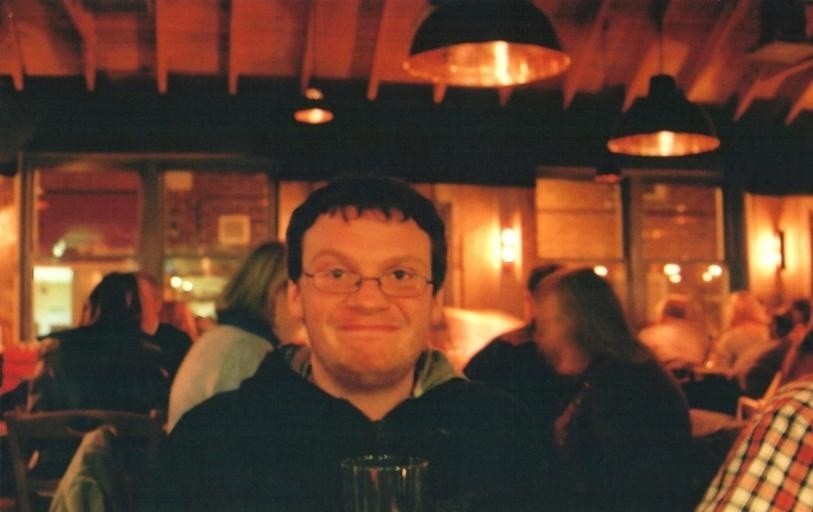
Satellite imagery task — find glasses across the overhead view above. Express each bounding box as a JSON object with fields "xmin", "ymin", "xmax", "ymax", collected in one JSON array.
[{"xmin": 300, "ymin": 266, "xmax": 432, "ymax": 299}]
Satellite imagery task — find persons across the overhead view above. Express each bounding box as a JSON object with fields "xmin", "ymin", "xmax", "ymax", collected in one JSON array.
[
  {"xmin": 145, "ymin": 177, "xmax": 570, "ymax": 509},
  {"xmin": 24, "ymin": 237, "xmax": 305, "ymax": 479},
  {"xmin": 463, "ymin": 260, "xmax": 813, "ymax": 510}
]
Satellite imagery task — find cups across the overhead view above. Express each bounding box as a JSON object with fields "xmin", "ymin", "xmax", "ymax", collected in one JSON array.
[{"xmin": 339, "ymin": 453, "xmax": 428, "ymax": 512}]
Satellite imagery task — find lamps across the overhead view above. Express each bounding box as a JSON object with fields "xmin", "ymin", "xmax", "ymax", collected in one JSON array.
[
  {"xmin": 291, "ymin": 1, "xmax": 336, "ymax": 126},
  {"xmin": 605, "ymin": 1, "xmax": 723, "ymax": 160},
  {"xmin": 404, "ymin": 0, "xmax": 570, "ymax": 88}
]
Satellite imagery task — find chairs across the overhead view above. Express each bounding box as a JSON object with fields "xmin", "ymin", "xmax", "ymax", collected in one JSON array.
[{"xmin": 3, "ymin": 399, "xmax": 169, "ymax": 512}]
